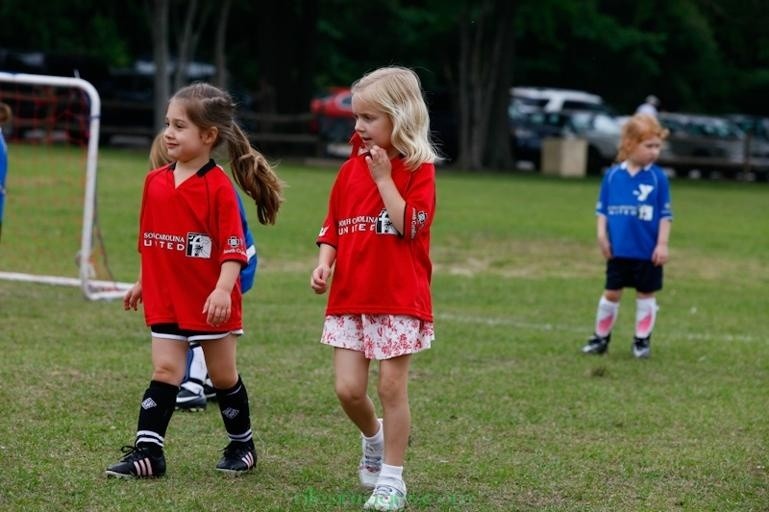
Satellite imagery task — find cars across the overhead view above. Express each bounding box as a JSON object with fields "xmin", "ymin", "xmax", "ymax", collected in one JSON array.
[
  {"xmin": 508, "ymin": 84, "xmax": 769, "ymax": 183},
  {"xmin": 309, "ymin": 85, "xmax": 358, "ymax": 136}
]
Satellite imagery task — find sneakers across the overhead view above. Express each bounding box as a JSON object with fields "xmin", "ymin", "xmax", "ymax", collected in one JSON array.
[
  {"xmin": 632, "ymin": 333, "xmax": 652, "ymax": 357},
  {"xmin": 214, "ymin": 437, "xmax": 258, "ymax": 473},
  {"xmin": 104, "ymin": 444, "xmax": 168, "ymax": 482},
  {"xmin": 580, "ymin": 331, "xmax": 611, "ymax": 354},
  {"xmin": 202, "ymin": 383, "xmax": 216, "ymax": 399},
  {"xmin": 357, "ymin": 416, "xmax": 385, "ymax": 489},
  {"xmin": 172, "ymin": 386, "xmax": 207, "ymax": 412},
  {"xmin": 363, "ymin": 474, "xmax": 406, "ymax": 512}
]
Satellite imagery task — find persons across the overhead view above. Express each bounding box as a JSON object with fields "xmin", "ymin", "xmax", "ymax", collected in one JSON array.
[
  {"xmin": 308, "ymin": 67, "xmax": 446, "ymax": 512},
  {"xmin": 578, "ymin": 118, "xmax": 674, "ymax": 358},
  {"xmin": 146, "ymin": 127, "xmax": 256, "ymax": 408},
  {"xmin": 104, "ymin": 82, "xmax": 283, "ymax": 478},
  {"xmin": 0, "ymin": 102, "xmax": 12, "ymax": 238},
  {"xmin": 636, "ymin": 96, "xmax": 658, "ymax": 117}
]
